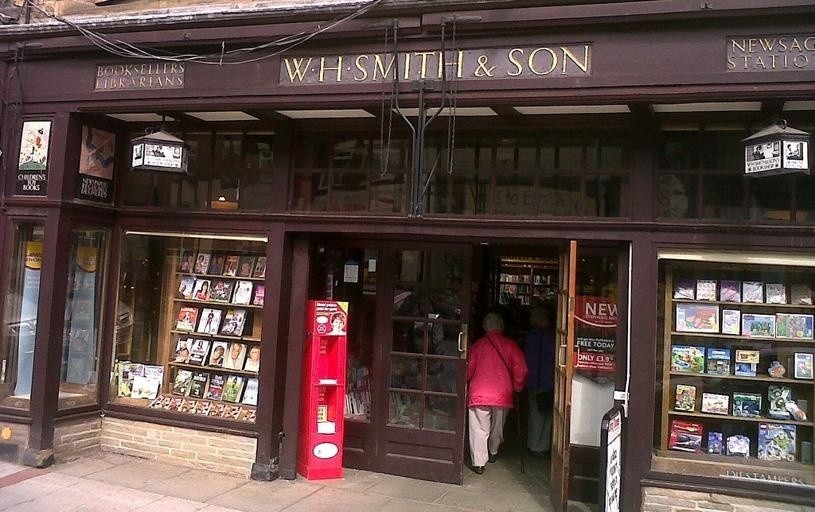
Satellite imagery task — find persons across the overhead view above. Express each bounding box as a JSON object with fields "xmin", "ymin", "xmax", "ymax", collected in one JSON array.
[
  {"xmin": 524, "ymin": 316, "xmax": 555, "ymax": 463},
  {"xmin": 327, "ymin": 312, "xmax": 346, "ymax": 334},
  {"xmin": 467, "ymin": 314, "xmax": 529, "ymax": 474}
]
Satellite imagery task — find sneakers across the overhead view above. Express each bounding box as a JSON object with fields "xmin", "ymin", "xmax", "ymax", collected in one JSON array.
[{"xmin": 531, "ymin": 450, "xmax": 549, "ymax": 458}]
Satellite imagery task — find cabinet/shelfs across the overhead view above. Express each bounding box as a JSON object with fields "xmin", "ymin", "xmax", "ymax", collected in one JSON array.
[
  {"xmin": 657, "ymin": 265, "xmax": 815, "ymax": 472},
  {"xmin": 489, "ymin": 257, "xmax": 559, "ymax": 330},
  {"xmin": 161, "ymin": 242, "xmax": 267, "ymax": 408}
]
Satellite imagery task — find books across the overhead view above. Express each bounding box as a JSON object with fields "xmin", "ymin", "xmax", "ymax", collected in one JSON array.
[
  {"xmin": 666, "ymin": 277, "xmax": 815, "ymax": 462},
  {"xmin": 112, "ymin": 238, "xmax": 268, "ymax": 423},
  {"xmin": 345, "ymin": 365, "xmax": 373, "ymax": 418}
]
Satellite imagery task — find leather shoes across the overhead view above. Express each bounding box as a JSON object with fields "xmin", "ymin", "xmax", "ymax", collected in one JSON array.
[
  {"xmin": 471, "ymin": 466, "xmax": 484, "ymax": 474},
  {"xmin": 489, "ymin": 449, "xmax": 501, "ymax": 463}
]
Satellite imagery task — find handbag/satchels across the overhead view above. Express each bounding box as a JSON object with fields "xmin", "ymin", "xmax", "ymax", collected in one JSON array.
[{"xmin": 536, "ymin": 391, "xmax": 554, "ymax": 412}]
